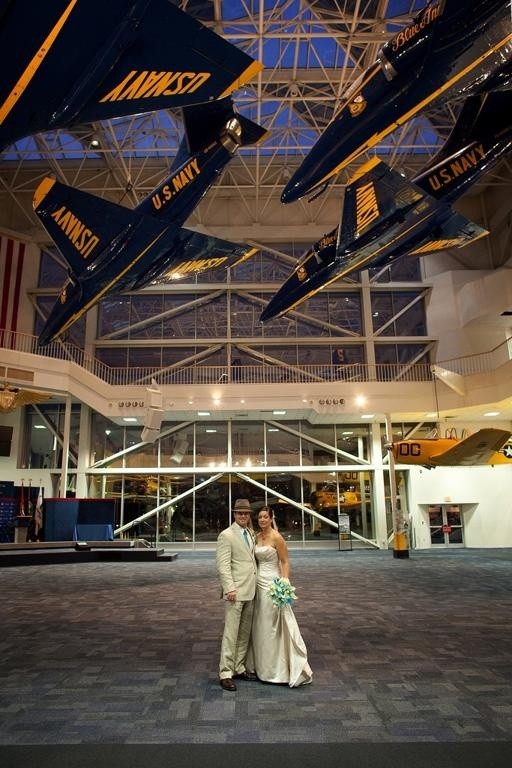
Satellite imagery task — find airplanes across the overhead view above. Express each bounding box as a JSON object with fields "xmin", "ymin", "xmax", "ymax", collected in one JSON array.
[
  {"xmin": 257, "ymin": 58, "xmax": 512, "ymax": 324},
  {"xmin": 306, "ymin": 489, "xmax": 400, "ymax": 512},
  {"xmin": 102, "ymin": 480, "xmax": 220, "ymax": 503},
  {"xmin": 0, "ymin": 1, "xmax": 266, "ymax": 149},
  {"xmin": 279, "ymin": 0, "xmax": 512, "ymax": 218},
  {"xmin": 383, "ymin": 427, "xmax": 512, "ymax": 467},
  {"xmin": 30, "ymin": 101, "xmax": 267, "ymax": 348}
]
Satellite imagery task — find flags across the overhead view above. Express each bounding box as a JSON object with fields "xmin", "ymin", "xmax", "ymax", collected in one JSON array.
[
  {"xmin": 34, "ymin": 486, "xmax": 44, "ymax": 529},
  {"xmin": 20, "ymin": 489, "xmax": 25, "ymax": 517},
  {"xmin": 28, "ymin": 498, "xmax": 35, "ymax": 522}
]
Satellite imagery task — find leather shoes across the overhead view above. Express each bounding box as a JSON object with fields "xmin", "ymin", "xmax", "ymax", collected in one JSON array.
[
  {"xmin": 232, "ymin": 671, "xmax": 257, "ymax": 681},
  {"xmin": 220, "ymin": 679, "xmax": 237, "ymax": 691}
]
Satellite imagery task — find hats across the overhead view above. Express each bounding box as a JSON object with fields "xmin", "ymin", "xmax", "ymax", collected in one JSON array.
[{"xmin": 231, "ymin": 499, "xmax": 253, "ymax": 513}]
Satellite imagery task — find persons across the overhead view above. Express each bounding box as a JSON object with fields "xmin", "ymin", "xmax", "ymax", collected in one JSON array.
[
  {"xmin": 253, "ymin": 507, "xmax": 313, "ymax": 687},
  {"xmin": 214, "ymin": 498, "xmax": 258, "ymax": 692}
]
